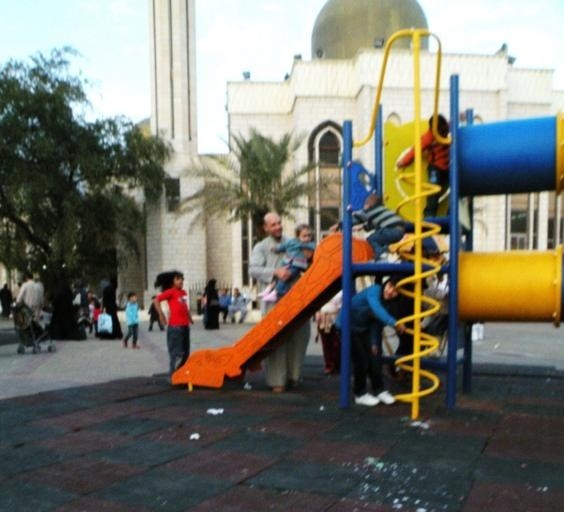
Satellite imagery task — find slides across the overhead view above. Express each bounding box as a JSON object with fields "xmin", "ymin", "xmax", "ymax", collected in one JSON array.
[{"xmin": 171, "ymin": 233, "xmax": 372, "ymax": 388}]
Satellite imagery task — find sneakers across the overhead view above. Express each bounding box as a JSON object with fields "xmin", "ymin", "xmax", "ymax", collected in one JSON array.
[
  {"xmin": 377, "ymin": 390, "xmax": 395, "ymax": 405},
  {"xmin": 354, "ymin": 394, "xmax": 379, "ymax": 406},
  {"xmin": 378, "ymin": 251, "xmax": 403, "ymax": 265}
]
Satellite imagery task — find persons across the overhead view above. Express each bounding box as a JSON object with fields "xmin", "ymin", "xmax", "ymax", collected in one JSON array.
[
  {"xmin": 0, "ymin": 271, "xmax": 247, "ymax": 377},
  {"xmin": 249, "ymin": 212, "xmax": 311, "ymax": 393},
  {"xmin": 331, "ymin": 195, "xmax": 405, "ymax": 264},
  {"xmin": 314, "ymin": 289, "xmax": 343, "ymax": 375},
  {"xmin": 352, "ymin": 277, "xmax": 406, "ymax": 407},
  {"xmin": 395, "ymin": 116, "xmax": 450, "ymax": 219},
  {"xmin": 256, "ymin": 225, "xmax": 316, "ymax": 302}
]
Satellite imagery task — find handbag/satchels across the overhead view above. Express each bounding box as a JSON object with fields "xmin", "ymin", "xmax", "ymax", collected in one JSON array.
[{"xmin": 96, "ymin": 313, "xmax": 114, "ymax": 336}]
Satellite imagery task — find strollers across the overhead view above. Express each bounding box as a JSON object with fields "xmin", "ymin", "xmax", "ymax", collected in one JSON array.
[{"xmin": 9, "ymin": 300, "xmax": 58, "ymax": 354}]
